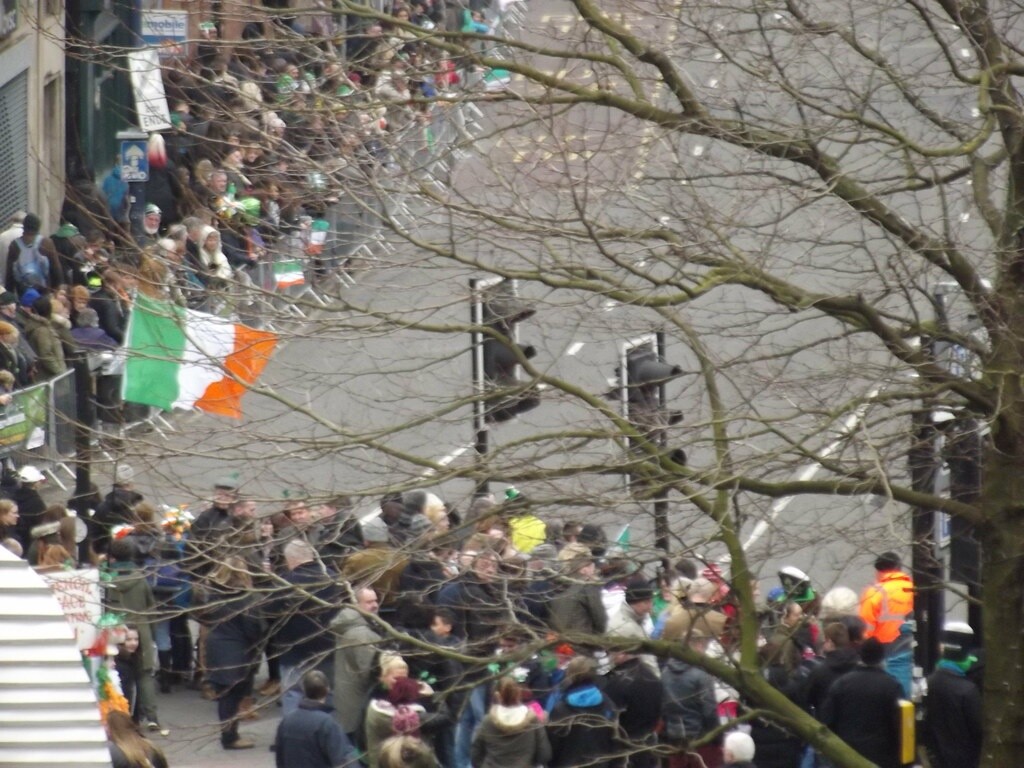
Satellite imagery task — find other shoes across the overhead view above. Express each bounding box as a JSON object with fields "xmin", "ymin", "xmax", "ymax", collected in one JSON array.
[
  {"xmin": 222, "ymin": 734, "xmax": 254, "ymax": 749},
  {"xmin": 148, "ymin": 719, "xmax": 161, "ymax": 730},
  {"xmin": 200, "ymin": 682, "xmax": 216, "ymax": 699},
  {"xmin": 261, "ymin": 675, "xmax": 281, "ymax": 695}
]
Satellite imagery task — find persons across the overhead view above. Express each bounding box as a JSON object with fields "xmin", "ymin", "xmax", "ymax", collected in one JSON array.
[
  {"xmin": 107, "ymin": 710, "xmax": 170, "ymax": 768},
  {"xmin": 0, "ymin": 131, "xmax": 227, "ymax": 403},
  {"xmin": 0, "ymin": 464, "xmax": 985, "ymax": 768},
  {"xmin": 115, "ymin": 7, "xmax": 490, "ymax": 276}
]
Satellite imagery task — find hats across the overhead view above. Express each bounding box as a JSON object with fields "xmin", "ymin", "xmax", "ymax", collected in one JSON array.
[
  {"xmin": 388, "ymin": 676, "xmax": 417, "ymax": 703},
  {"xmin": 19, "ymin": 465, "xmax": 46, "ymax": 484},
  {"xmin": 818, "ymin": 587, "xmax": 859, "ymax": 619},
  {"xmin": 32, "ymin": 298, "xmax": 51, "ymax": 313},
  {"xmin": 212, "ymin": 476, "xmax": 238, "ymax": 492},
  {"xmin": 625, "ymin": 577, "xmax": 652, "ymax": 603},
  {"xmin": 69, "ymin": 285, "xmax": 90, "ymax": 300},
  {"xmin": 687, "ymin": 577, "xmax": 713, "ymax": 604},
  {"xmin": 113, "ymin": 464, "xmax": 133, "ymax": 485},
  {"xmin": 391, "ymin": 705, "xmax": 420, "ymax": 737},
  {"xmin": 21, "ymin": 288, "xmax": 41, "ymax": 307},
  {"xmin": 0, "ymin": 292, "xmax": 20, "ymax": 305}
]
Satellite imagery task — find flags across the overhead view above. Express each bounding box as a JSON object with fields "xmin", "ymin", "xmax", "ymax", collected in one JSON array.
[
  {"xmin": 303, "ymin": 219, "xmax": 330, "ymax": 256},
  {"xmin": 270, "ymin": 261, "xmax": 306, "ymax": 288},
  {"xmin": 118, "ymin": 290, "xmax": 279, "ymax": 420}
]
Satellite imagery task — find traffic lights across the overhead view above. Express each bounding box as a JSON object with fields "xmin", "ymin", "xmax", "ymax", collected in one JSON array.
[
  {"xmin": 623, "ymin": 334, "xmax": 688, "ymax": 501},
  {"xmin": 471, "ymin": 276, "xmax": 542, "ymax": 429}
]
